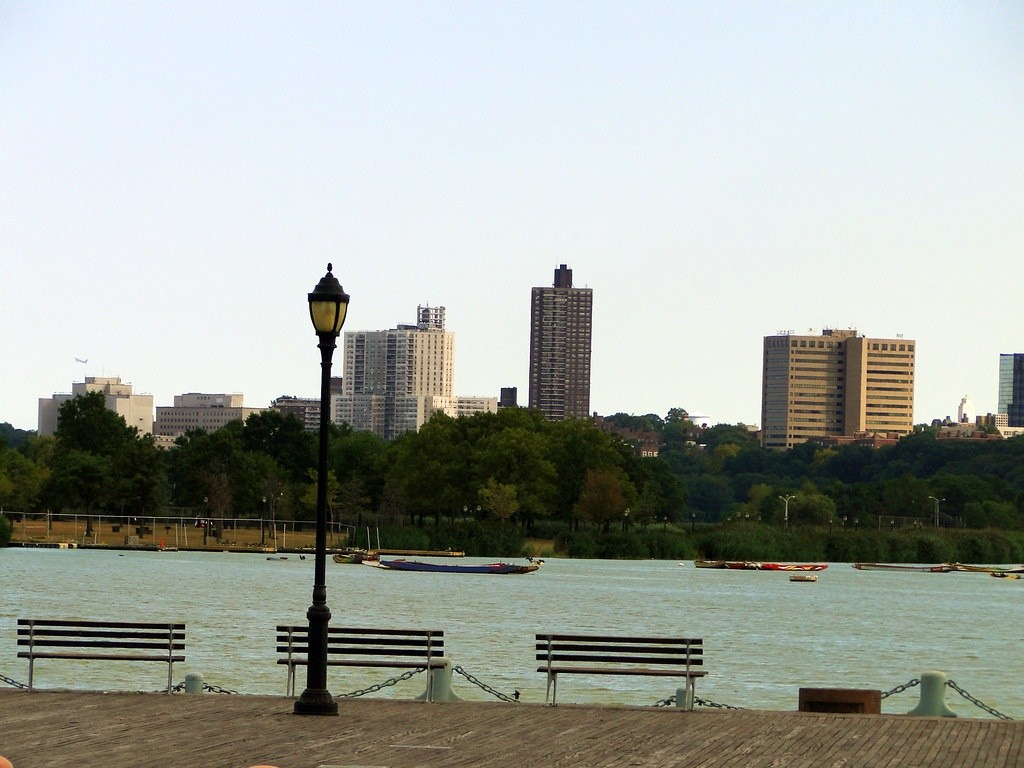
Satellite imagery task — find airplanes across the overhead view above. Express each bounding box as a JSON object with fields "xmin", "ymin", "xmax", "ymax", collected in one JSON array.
[{"xmin": 75, "ymin": 358, "xmax": 88, "ymax": 363}]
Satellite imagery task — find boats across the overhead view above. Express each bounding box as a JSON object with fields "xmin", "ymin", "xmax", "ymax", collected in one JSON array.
[
  {"xmin": 990, "ymin": 572, "xmax": 1021, "ymax": 579},
  {"xmin": 852, "ymin": 564, "xmax": 954, "ymax": 573},
  {"xmin": 950, "ymin": 564, "xmax": 1023, "ymax": 573},
  {"xmin": 694, "ymin": 560, "xmax": 828, "ymax": 570},
  {"xmin": 378, "ymin": 559, "xmax": 539, "ymax": 574},
  {"xmin": 353, "ymin": 551, "xmax": 378, "ymax": 560},
  {"xmin": 789, "ymin": 576, "xmax": 818, "ymax": 582},
  {"xmin": 333, "ymin": 555, "xmax": 363, "ymax": 564}
]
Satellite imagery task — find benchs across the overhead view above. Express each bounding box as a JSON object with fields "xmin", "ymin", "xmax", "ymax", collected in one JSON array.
[
  {"xmin": 14, "ymin": 619, "xmax": 186, "ymax": 694},
  {"xmin": 534, "ymin": 631, "xmax": 707, "ymax": 711},
  {"xmin": 274, "ymin": 624, "xmax": 445, "ymax": 702}
]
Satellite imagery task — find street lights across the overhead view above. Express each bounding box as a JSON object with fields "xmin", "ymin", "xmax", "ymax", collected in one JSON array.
[
  {"xmin": 262, "ymin": 496, "xmax": 267, "ymax": 544},
  {"xmin": 919, "ymin": 521, "xmax": 922, "ymax": 531},
  {"xmin": 855, "ymin": 519, "xmax": 858, "ymax": 531},
  {"xmin": 779, "ymin": 496, "xmax": 795, "ymax": 528},
  {"xmin": 663, "ymin": 515, "xmax": 667, "ymax": 527},
  {"xmin": 758, "ymin": 514, "xmax": 761, "ymax": 523},
  {"xmin": 745, "ymin": 512, "xmax": 749, "ymax": 521},
  {"xmin": 692, "ymin": 513, "xmax": 695, "ymax": 527},
  {"xmin": 463, "ymin": 505, "xmax": 467, "ymax": 522},
  {"xmin": 844, "ymin": 516, "xmax": 847, "ymax": 532},
  {"xmin": 204, "ymin": 496, "xmax": 208, "ymax": 545},
  {"xmin": 292, "ymin": 263, "xmax": 350, "ymax": 715},
  {"xmin": 727, "ymin": 516, "xmax": 730, "ymax": 523},
  {"xmin": 737, "ymin": 511, "xmax": 740, "ymax": 521},
  {"xmin": 913, "ymin": 520, "xmax": 917, "ymax": 528},
  {"xmin": 891, "ymin": 520, "xmax": 894, "ymax": 530},
  {"xmin": 624, "ymin": 510, "xmax": 628, "ymax": 530},
  {"xmin": 477, "ymin": 505, "xmax": 481, "ymax": 520},
  {"xmin": 929, "ymin": 496, "xmax": 945, "ymax": 528},
  {"xmin": 829, "ymin": 519, "xmax": 832, "ymax": 533}
]
